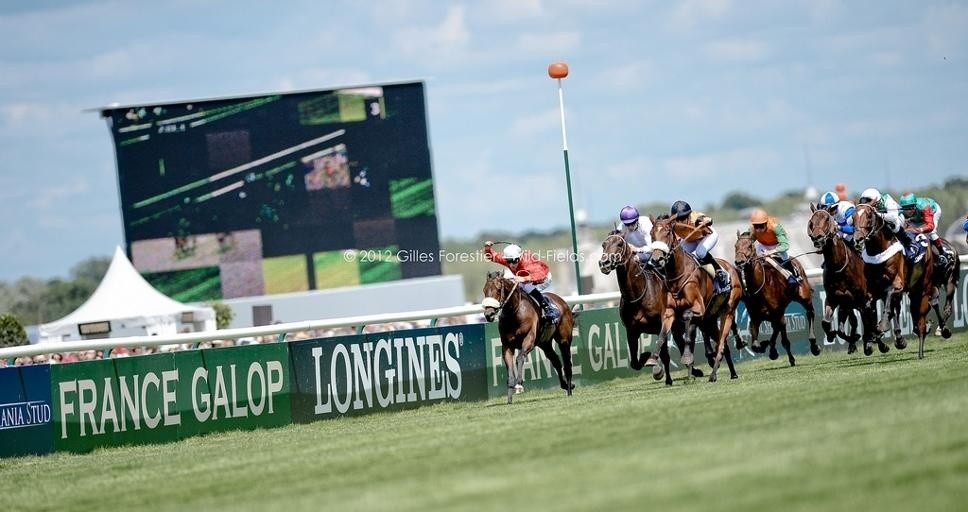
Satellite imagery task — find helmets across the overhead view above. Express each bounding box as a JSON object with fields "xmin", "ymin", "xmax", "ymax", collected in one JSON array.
[
  {"xmin": 820, "ymin": 192, "xmax": 840, "ymax": 206},
  {"xmin": 900, "ymin": 192, "xmax": 917, "ymax": 207},
  {"xmin": 862, "ymin": 189, "xmax": 881, "ymax": 205},
  {"xmin": 502, "ymin": 244, "xmax": 522, "ymax": 258},
  {"xmin": 671, "ymin": 201, "xmax": 692, "ymax": 217},
  {"xmin": 751, "ymin": 210, "xmax": 767, "ymax": 224},
  {"xmin": 620, "ymin": 206, "xmax": 639, "ymax": 224}
]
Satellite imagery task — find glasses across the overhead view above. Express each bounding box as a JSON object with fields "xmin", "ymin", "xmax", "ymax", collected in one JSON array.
[
  {"xmin": 506, "ymin": 258, "xmax": 519, "ymax": 264},
  {"xmin": 753, "ymin": 224, "xmax": 764, "ymax": 228},
  {"xmin": 859, "ymin": 198, "xmax": 871, "ymax": 202},
  {"xmin": 624, "ymin": 221, "xmax": 636, "ymax": 227}
]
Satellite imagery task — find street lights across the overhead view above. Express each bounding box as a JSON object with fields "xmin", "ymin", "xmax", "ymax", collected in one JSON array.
[{"xmin": 545, "ymin": 58, "xmax": 587, "ymax": 310}]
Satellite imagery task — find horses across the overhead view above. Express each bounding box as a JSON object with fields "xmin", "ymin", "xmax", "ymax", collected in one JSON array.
[
  {"xmin": 735, "ymin": 225, "xmax": 822, "ymax": 366},
  {"xmin": 483, "ymin": 269, "xmax": 583, "ymax": 405},
  {"xmin": 596, "ymin": 226, "xmax": 747, "ymax": 384},
  {"xmin": 806, "ymin": 202, "xmax": 961, "ymax": 358}
]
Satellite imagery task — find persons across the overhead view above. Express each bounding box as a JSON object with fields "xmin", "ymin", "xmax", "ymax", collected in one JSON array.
[
  {"xmin": 670, "ymin": 201, "xmax": 731, "ymax": 290},
  {"xmin": 899, "ymin": 191, "xmax": 949, "ymax": 266},
  {"xmin": 484, "ymin": 241, "xmax": 561, "ymax": 322},
  {"xmin": 858, "ymin": 188, "xmax": 920, "ymax": 259},
  {"xmin": 816, "ymin": 192, "xmax": 856, "ymax": 246},
  {"xmin": 748, "ymin": 208, "xmax": 803, "ymax": 283},
  {"xmin": 0, "ymin": 312, "xmax": 490, "ymax": 370},
  {"xmin": 608, "ymin": 206, "xmax": 654, "ymax": 263}
]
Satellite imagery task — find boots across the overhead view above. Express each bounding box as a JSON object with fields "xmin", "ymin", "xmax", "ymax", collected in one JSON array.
[
  {"xmin": 932, "ymin": 238, "xmax": 948, "ymax": 267},
  {"xmin": 702, "ymin": 252, "xmax": 727, "ymax": 289},
  {"xmin": 529, "ymin": 288, "xmax": 553, "ymax": 321},
  {"xmin": 784, "ymin": 259, "xmax": 803, "ymax": 284}
]
